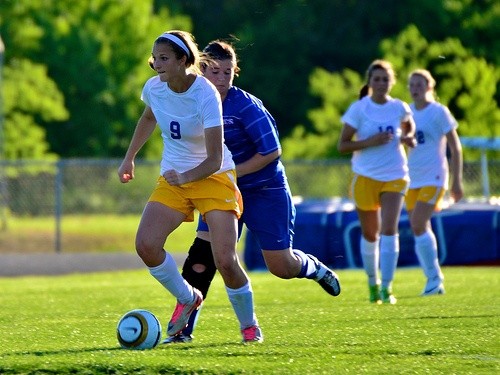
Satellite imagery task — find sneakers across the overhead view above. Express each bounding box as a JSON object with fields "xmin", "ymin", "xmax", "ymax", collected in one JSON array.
[
  {"xmin": 241, "ymin": 326, "xmax": 263, "ymax": 342},
  {"xmin": 166, "ymin": 287, "xmax": 203, "ymax": 335},
  {"xmin": 307, "ymin": 253, "xmax": 340, "ymax": 296},
  {"xmin": 164, "ymin": 334, "xmax": 192, "ymax": 343}
]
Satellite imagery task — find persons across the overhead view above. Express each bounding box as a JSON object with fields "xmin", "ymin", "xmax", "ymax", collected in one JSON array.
[
  {"xmin": 118, "ymin": 30, "xmax": 265, "ymax": 343},
  {"xmin": 405, "ymin": 70, "xmax": 464, "ymax": 295},
  {"xmin": 338, "ymin": 60, "xmax": 417, "ymax": 304},
  {"xmin": 163, "ymin": 41, "xmax": 341, "ymax": 342}
]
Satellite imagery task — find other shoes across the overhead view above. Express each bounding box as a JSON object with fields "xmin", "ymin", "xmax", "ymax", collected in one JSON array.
[
  {"xmin": 423, "ymin": 283, "xmax": 444, "ymax": 295},
  {"xmin": 370, "ymin": 282, "xmax": 383, "ymax": 304},
  {"xmin": 381, "ymin": 283, "xmax": 396, "ymax": 304}
]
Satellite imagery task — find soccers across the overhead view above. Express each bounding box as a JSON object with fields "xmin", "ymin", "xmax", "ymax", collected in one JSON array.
[{"xmin": 116, "ymin": 309, "xmax": 162, "ymax": 350}]
